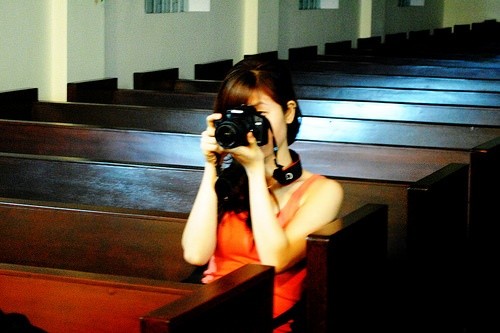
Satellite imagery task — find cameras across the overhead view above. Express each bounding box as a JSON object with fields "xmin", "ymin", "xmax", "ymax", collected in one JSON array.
[{"xmin": 213, "ymin": 104, "xmax": 270, "ymax": 150}]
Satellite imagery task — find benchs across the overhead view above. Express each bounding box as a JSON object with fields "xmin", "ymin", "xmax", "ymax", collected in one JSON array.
[{"xmin": 1, "ymin": 24, "xmax": 500, "ymax": 333}]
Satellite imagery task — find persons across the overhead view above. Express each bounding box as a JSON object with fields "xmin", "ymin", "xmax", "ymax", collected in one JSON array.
[{"xmin": 180, "ymin": 59, "xmax": 345, "ymax": 333}]
